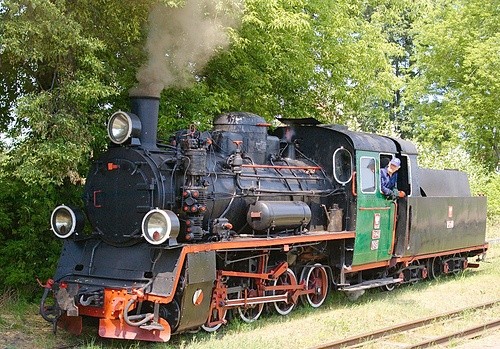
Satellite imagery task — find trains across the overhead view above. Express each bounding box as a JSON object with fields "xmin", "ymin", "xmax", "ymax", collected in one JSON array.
[{"xmin": 38, "ymin": 94, "xmax": 489, "ymax": 344}]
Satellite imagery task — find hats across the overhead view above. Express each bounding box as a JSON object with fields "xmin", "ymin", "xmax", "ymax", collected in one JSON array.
[{"xmin": 388, "ymin": 157, "xmax": 401, "ymax": 167}]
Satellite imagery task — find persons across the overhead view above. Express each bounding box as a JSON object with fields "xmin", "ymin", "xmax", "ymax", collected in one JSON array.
[
  {"xmin": 363, "ymin": 158, "xmax": 375, "ymax": 192},
  {"xmin": 379, "ymin": 157, "xmax": 407, "ymax": 198}
]
